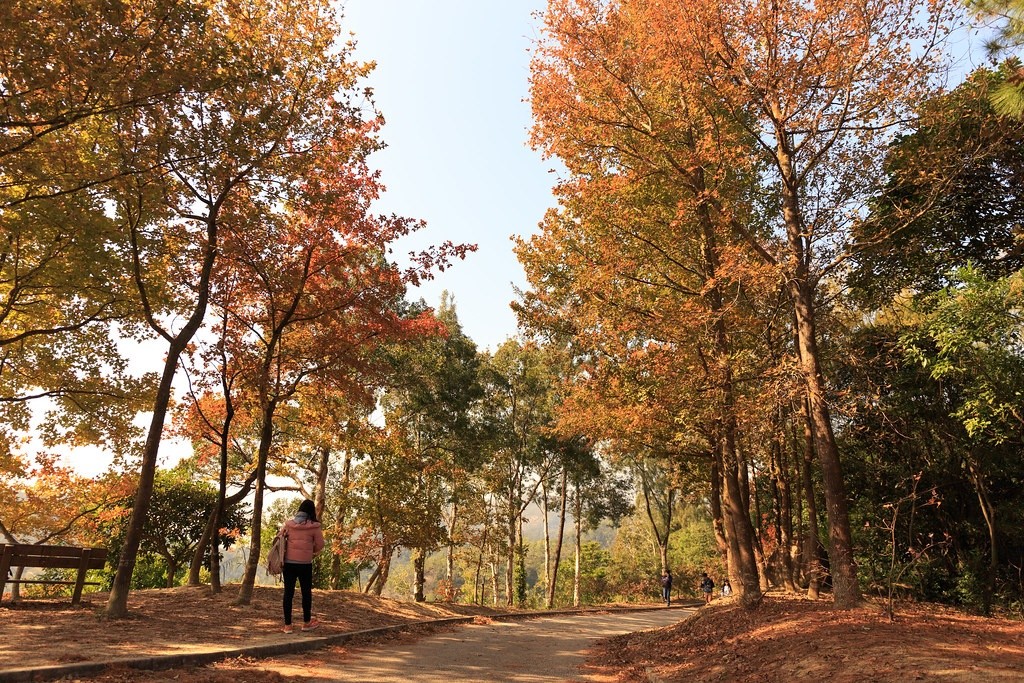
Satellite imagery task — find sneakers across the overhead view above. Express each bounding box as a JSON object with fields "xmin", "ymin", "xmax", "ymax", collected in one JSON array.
[
  {"xmin": 281, "ymin": 624, "xmax": 293, "ymax": 633},
  {"xmin": 302, "ymin": 621, "xmax": 320, "ymax": 631}
]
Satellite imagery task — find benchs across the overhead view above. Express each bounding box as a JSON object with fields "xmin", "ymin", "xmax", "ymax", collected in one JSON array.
[{"xmin": 0, "ymin": 543, "xmax": 109, "ymax": 605}]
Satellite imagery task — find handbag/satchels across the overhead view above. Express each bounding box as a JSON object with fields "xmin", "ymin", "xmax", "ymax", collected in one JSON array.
[{"xmin": 266, "ymin": 532, "xmax": 286, "ymax": 575}]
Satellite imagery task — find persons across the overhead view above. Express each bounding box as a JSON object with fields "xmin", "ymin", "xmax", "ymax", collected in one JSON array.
[
  {"xmin": 721, "ymin": 580, "xmax": 732, "ymax": 596},
  {"xmin": 280, "ymin": 499, "xmax": 324, "ymax": 634},
  {"xmin": 700, "ymin": 573, "xmax": 715, "ymax": 604},
  {"xmin": 662, "ymin": 570, "xmax": 672, "ymax": 606}
]
